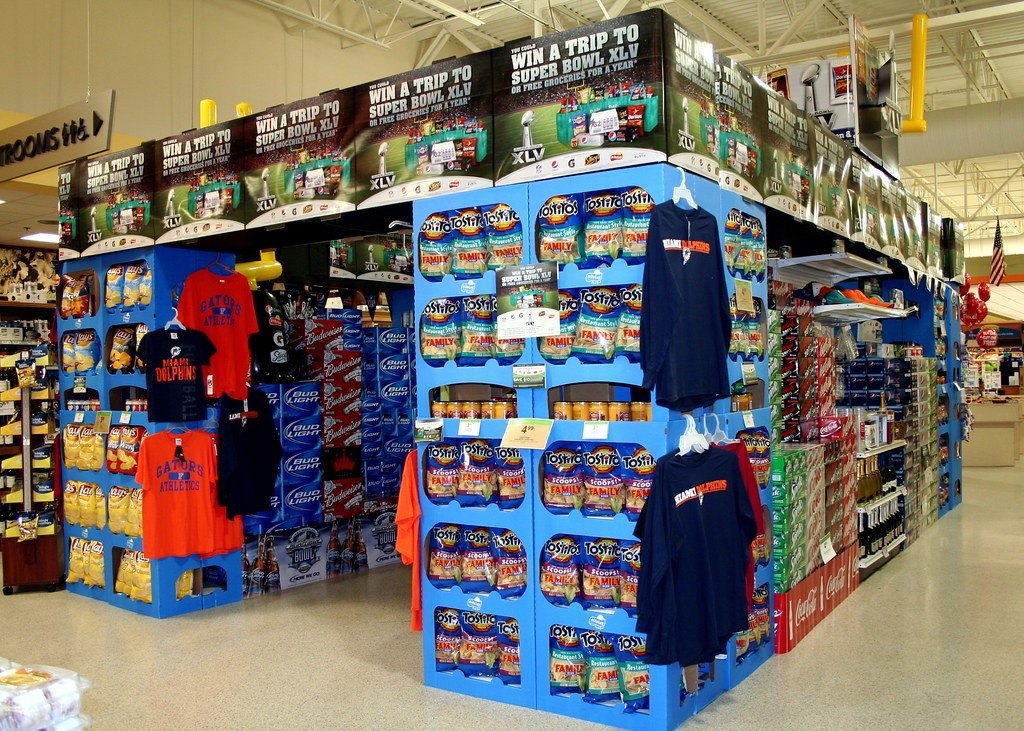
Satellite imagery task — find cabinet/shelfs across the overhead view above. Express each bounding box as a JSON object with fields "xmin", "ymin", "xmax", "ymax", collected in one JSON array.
[
  {"xmin": 771, "ymin": 253, "xmax": 909, "ymax": 321},
  {"xmin": 849, "ymin": 438, "xmax": 908, "ymax": 569},
  {"xmin": 0, "ymin": 301, "xmax": 63, "ymax": 594}
]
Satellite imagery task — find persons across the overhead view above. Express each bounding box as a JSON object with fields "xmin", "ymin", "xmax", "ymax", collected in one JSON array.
[{"xmin": 998, "ymin": 355, "xmax": 1012, "ymax": 385}]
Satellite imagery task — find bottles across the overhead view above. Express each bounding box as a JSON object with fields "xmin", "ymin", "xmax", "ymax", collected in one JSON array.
[
  {"xmin": 249, "ymin": 533, "xmax": 266, "ymax": 598},
  {"xmin": 353, "ymin": 522, "xmax": 369, "ymax": 573},
  {"xmin": 342, "ymin": 525, "xmax": 355, "ymax": 576},
  {"xmin": 0, "ymin": 415, "xmax": 13, "ymax": 446},
  {"xmin": 326, "ymin": 519, "xmax": 342, "ymax": 579},
  {"xmin": 0, "ymin": 367, "xmax": 17, "ymax": 392},
  {"xmin": 857, "ymin": 451, "xmax": 897, "ymax": 507},
  {"xmin": 264, "ymin": 537, "xmax": 281, "ymax": 594},
  {"xmin": 858, "ymin": 497, "xmax": 904, "ymax": 559},
  {"xmin": 0, "ymin": 469, "xmax": 16, "ymax": 491},
  {"xmin": 241, "ymin": 537, "xmax": 251, "ymax": 599},
  {"xmin": 0, "ymin": 503, "xmax": 16, "ymax": 535}
]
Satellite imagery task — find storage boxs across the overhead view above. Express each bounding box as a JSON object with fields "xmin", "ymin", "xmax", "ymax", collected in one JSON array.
[{"xmin": 57, "ymin": 8, "xmax": 962, "ymax": 731}]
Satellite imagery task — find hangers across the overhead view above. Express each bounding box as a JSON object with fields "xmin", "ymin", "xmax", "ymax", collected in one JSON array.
[
  {"xmin": 679, "ymin": 413, "xmax": 739, "ymax": 455},
  {"xmin": 164, "ymin": 305, "xmax": 187, "ymax": 332},
  {"xmin": 205, "ymin": 250, "xmax": 235, "ymax": 276},
  {"xmin": 674, "ymin": 167, "xmax": 699, "ymax": 210},
  {"xmin": 166, "ymin": 420, "xmax": 190, "ymax": 434}
]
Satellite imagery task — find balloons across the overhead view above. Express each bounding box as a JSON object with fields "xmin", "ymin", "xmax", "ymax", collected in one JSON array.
[
  {"xmin": 977, "ymin": 328, "xmax": 998, "ymax": 349},
  {"xmin": 958, "ymin": 271, "xmax": 991, "ymax": 327}
]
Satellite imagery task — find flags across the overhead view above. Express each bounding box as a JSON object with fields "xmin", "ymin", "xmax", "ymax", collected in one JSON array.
[{"xmin": 990, "ymin": 218, "xmax": 1006, "ymax": 286}]
